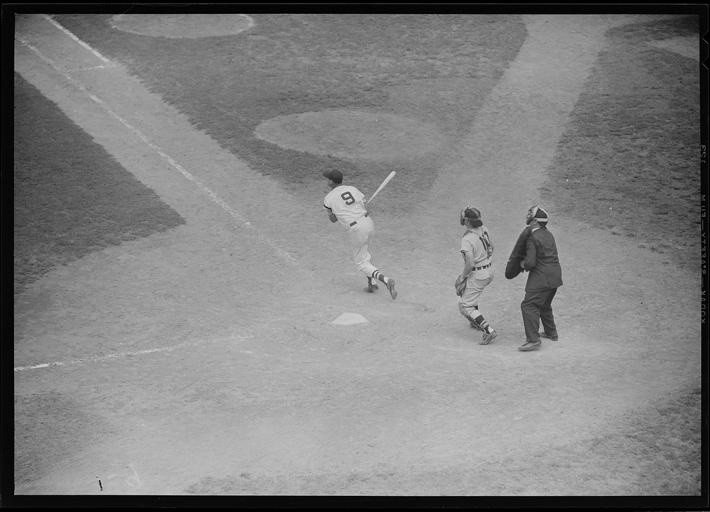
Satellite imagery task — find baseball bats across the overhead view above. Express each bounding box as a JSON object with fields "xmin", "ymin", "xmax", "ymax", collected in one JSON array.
[{"xmin": 366, "ymin": 171, "xmax": 396, "ymax": 203}]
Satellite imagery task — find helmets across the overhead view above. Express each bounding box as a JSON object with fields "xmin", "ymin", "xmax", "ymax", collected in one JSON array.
[
  {"xmin": 526, "ymin": 205, "xmax": 548, "ymax": 227},
  {"xmin": 460, "ymin": 205, "xmax": 483, "ymax": 227}
]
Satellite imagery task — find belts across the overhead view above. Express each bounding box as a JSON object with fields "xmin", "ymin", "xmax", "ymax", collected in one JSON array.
[
  {"xmin": 351, "ymin": 213, "xmax": 368, "ymax": 225},
  {"xmin": 473, "ymin": 263, "xmax": 492, "ymax": 271}
]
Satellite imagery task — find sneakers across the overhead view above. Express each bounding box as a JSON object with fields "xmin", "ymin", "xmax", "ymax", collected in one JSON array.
[
  {"xmin": 470, "ymin": 322, "xmax": 497, "ymax": 344},
  {"xmin": 520, "ymin": 332, "xmax": 558, "ymax": 351},
  {"xmin": 365, "ymin": 284, "xmax": 379, "ymax": 292},
  {"xmin": 387, "ymin": 279, "xmax": 397, "ymax": 299}
]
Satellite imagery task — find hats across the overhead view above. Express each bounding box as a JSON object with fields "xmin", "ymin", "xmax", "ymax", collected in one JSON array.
[{"xmin": 323, "ymin": 170, "xmax": 343, "ymax": 184}]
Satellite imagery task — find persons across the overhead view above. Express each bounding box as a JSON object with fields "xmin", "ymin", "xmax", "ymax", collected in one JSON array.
[
  {"xmin": 322, "ymin": 170, "xmax": 398, "ymax": 301},
  {"xmin": 517, "ymin": 205, "xmax": 564, "ymax": 352},
  {"xmin": 454, "ymin": 205, "xmax": 498, "ymax": 345}
]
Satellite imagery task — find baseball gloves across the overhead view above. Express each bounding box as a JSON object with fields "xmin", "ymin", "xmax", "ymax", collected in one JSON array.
[{"xmin": 455, "ymin": 274, "xmax": 467, "ymax": 296}]
